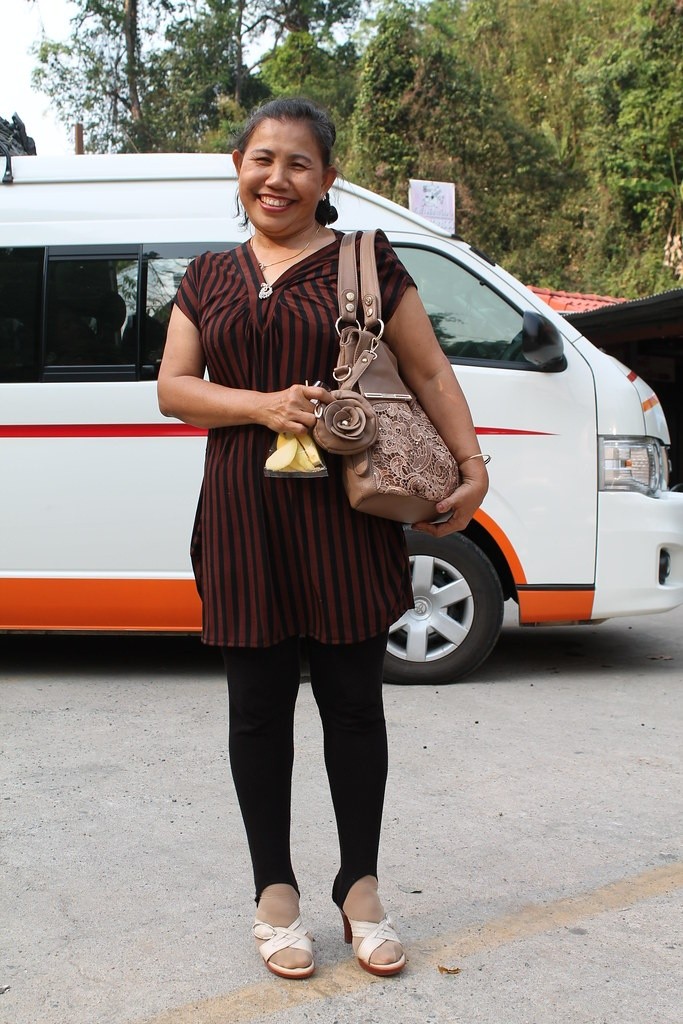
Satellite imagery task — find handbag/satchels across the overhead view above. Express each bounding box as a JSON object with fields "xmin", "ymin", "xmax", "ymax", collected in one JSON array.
[{"xmin": 313, "ymin": 228, "xmax": 462, "ymax": 525}]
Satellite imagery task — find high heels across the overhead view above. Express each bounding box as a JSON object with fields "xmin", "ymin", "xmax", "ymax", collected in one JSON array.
[
  {"xmin": 332, "ymin": 881, "xmax": 406, "ymax": 977},
  {"xmin": 250, "ymin": 910, "xmax": 316, "ymax": 980}
]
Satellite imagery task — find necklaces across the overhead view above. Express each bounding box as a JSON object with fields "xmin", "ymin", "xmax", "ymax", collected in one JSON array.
[{"xmin": 251, "ymin": 225, "xmax": 321, "ymax": 273}]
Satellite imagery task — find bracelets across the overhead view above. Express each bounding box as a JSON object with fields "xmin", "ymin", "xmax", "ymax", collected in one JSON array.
[{"xmin": 456, "ymin": 452, "xmax": 492, "ymax": 466}]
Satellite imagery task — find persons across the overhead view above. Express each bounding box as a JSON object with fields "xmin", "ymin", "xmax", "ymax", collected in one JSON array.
[
  {"xmin": 42, "ymin": 285, "xmax": 126, "ymax": 381},
  {"xmin": 157, "ymin": 99, "xmax": 492, "ymax": 980}
]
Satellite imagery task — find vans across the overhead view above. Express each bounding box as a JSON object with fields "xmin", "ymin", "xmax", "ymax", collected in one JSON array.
[{"xmin": 0, "ymin": 153, "xmax": 682, "ymax": 687}]
